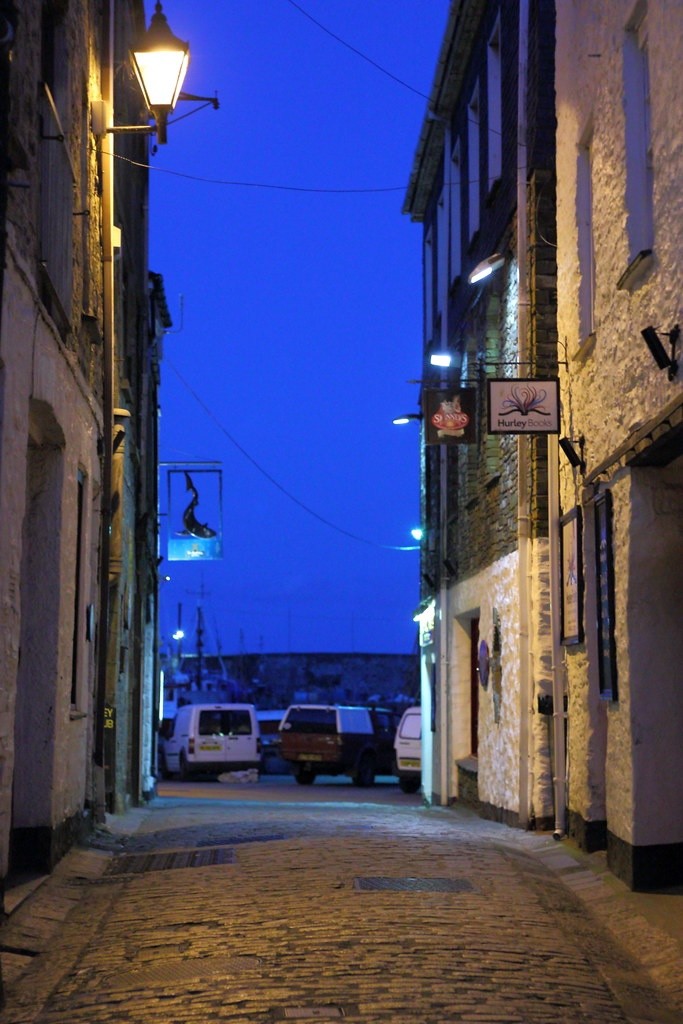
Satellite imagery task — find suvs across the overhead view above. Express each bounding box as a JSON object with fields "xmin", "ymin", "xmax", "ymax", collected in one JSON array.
[{"xmin": 280, "ymin": 705, "xmax": 395, "ymax": 788}]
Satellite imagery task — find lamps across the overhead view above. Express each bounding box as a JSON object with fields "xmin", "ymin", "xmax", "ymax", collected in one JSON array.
[
  {"xmin": 392, "ymin": 413, "xmax": 423, "ymax": 425},
  {"xmin": 124, "ymin": 0, "xmax": 194, "ymax": 146},
  {"xmin": 467, "ymin": 251, "xmax": 508, "ymax": 285},
  {"xmin": 430, "ymin": 352, "xmax": 461, "ymax": 368}
]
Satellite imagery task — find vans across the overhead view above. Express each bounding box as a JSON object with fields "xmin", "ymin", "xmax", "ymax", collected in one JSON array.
[
  {"xmin": 160, "ymin": 704, "xmax": 262, "ymax": 781},
  {"xmin": 395, "ymin": 706, "xmax": 423, "ymax": 793},
  {"xmin": 255, "ymin": 710, "xmax": 290, "ymax": 756}
]
